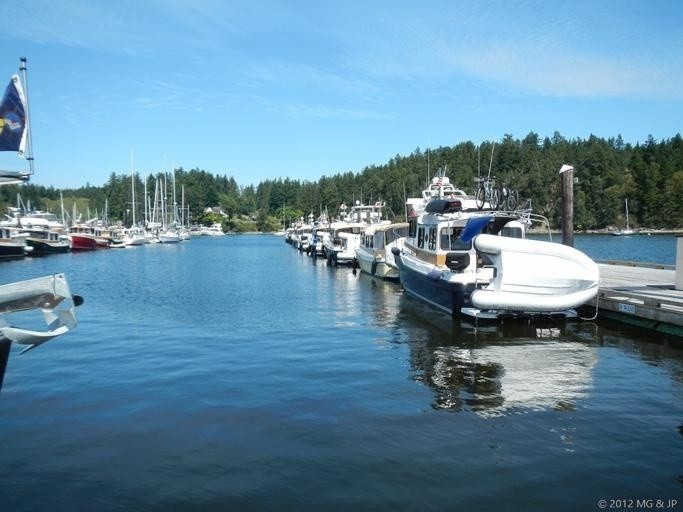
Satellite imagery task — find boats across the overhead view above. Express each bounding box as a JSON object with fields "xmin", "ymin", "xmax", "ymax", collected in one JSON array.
[
  {"xmin": 286, "ymin": 181, "xmax": 409, "ymax": 279},
  {"xmin": 189, "ymin": 223, "xmax": 225, "ymax": 236},
  {"xmin": 392, "ymin": 143, "xmax": 600, "ymax": 326},
  {"xmin": 0, "ymin": 193, "xmax": 126, "ymax": 258}
]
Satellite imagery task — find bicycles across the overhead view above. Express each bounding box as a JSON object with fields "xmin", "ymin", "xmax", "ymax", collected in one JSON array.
[{"xmin": 475, "ymin": 176, "xmax": 519, "ymax": 211}]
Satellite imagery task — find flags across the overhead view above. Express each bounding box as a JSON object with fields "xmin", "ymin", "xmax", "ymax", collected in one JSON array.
[{"xmin": 0, "ymin": 74, "xmax": 28, "ymax": 158}]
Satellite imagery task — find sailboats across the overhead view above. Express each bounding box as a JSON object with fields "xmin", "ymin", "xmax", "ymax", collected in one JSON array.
[{"xmin": 125, "ymin": 149, "xmax": 190, "ymax": 246}]
